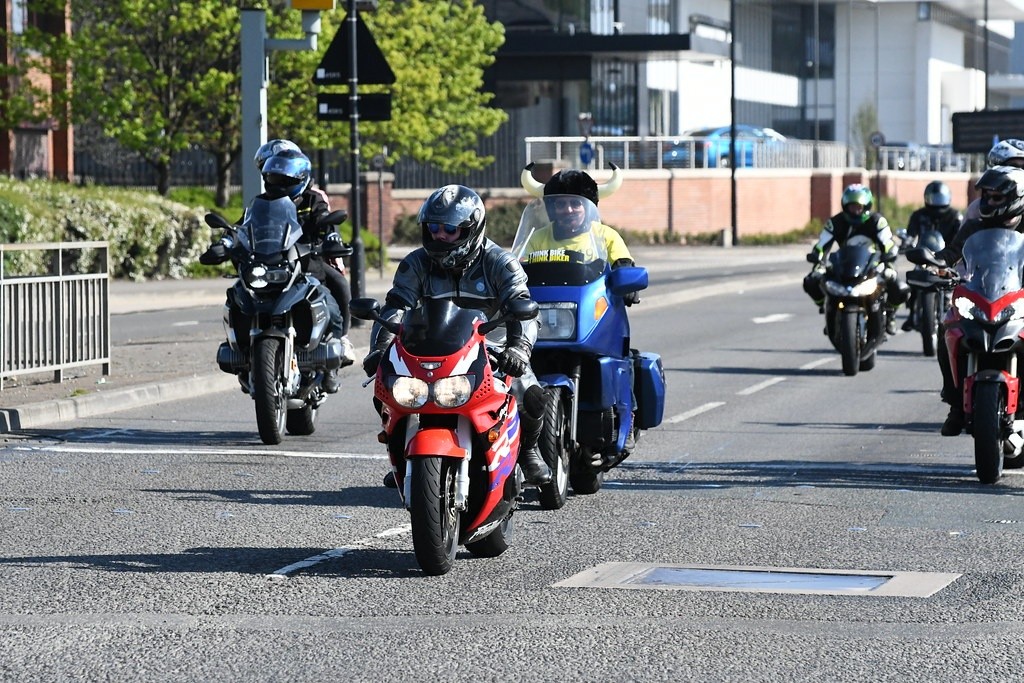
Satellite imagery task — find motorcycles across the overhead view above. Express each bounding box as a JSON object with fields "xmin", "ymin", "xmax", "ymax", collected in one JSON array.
[
  {"xmin": 904, "ymin": 227, "xmax": 1023, "ymax": 484},
  {"xmin": 893, "ymin": 227, "xmax": 962, "ymax": 358},
  {"xmin": 803, "ymin": 236, "xmax": 902, "ymax": 373},
  {"xmin": 341, "ymin": 290, "xmax": 528, "ymax": 579},
  {"xmin": 498, "ymin": 193, "xmax": 674, "ymax": 510},
  {"xmin": 198, "ymin": 209, "xmax": 359, "ymax": 444}
]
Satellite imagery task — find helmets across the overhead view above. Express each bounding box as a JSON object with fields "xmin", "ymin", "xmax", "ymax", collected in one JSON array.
[
  {"xmin": 975, "ymin": 165, "xmax": 1024, "ymax": 229},
  {"xmin": 924, "ymin": 182, "xmax": 951, "ymax": 214},
  {"xmin": 842, "ymin": 184, "xmax": 874, "ymax": 223},
  {"xmin": 254, "ymin": 139, "xmax": 302, "ymax": 173},
  {"xmin": 987, "ymin": 139, "xmax": 1024, "ymax": 168},
  {"xmin": 417, "ymin": 184, "xmax": 486, "ymax": 269},
  {"xmin": 262, "ymin": 148, "xmax": 311, "ymax": 199}
]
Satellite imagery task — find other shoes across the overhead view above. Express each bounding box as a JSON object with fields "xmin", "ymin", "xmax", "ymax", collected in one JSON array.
[
  {"xmin": 340, "ymin": 337, "xmax": 355, "ymax": 363},
  {"xmin": 238, "ymin": 372, "xmax": 249, "ymax": 394},
  {"xmin": 322, "ymin": 369, "xmax": 340, "ymax": 393},
  {"xmin": 887, "ymin": 321, "xmax": 897, "ymax": 335},
  {"xmin": 902, "ymin": 320, "xmax": 914, "ymax": 331},
  {"xmin": 941, "ymin": 403, "xmax": 965, "ymax": 436}
]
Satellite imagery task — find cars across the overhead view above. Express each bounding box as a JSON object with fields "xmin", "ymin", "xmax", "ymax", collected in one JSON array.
[
  {"xmin": 877, "ymin": 142, "xmax": 970, "ymax": 174},
  {"xmin": 660, "ymin": 125, "xmax": 792, "ymax": 171}
]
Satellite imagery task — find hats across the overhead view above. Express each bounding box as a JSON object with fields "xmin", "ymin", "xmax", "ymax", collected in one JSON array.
[{"xmin": 544, "ymin": 170, "xmax": 599, "ymax": 221}]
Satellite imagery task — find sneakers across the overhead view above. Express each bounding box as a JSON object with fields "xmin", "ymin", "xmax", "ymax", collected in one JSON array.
[{"xmin": 613, "ymin": 412, "xmax": 636, "ymax": 454}]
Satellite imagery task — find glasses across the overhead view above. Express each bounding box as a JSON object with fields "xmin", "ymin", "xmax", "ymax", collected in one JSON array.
[
  {"xmin": 554, "ymin": 199, "xmax": 583, "ymax": 209},
  {"xmin": 427, "ymin": 223, "xmax": 462, "ymax": 235},
  {"xmin": 984, "ymin": 194, "xmax": 1008, "ymax": 201}
]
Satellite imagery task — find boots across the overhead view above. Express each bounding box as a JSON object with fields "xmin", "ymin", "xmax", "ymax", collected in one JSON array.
[
  {"xmin": 518, "ymin": 408, "xmax": 549, "ymax": 485},
  {"xmin": 384, "ymin": 417, "xmax": 407, "ymax": 487}
]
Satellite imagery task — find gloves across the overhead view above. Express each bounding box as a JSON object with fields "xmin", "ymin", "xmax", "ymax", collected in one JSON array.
[
  {"xmin": 219, "ymin": 235, "xmax": 233, "ymax": 251},
  {"xmin": 623, "ymin": 291, "xmax": 639, "ymax": 307},
  {"xmin": 497, "ymin": 334, "xmax": 532, "ymax": 378},
  {"xmin": 363, "ymin": 342, "xmax": 389, "ymax": 377},
  {"xmin": 325, "ymin": 232, "xmax": 343, "ymax": 247}
]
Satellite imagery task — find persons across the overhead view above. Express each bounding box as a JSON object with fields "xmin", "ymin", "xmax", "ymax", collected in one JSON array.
[
  {"xmin": 364, "ymin": 185, "xmax": 554, "ymax": 489},
  {"xmin": 802, "ymin": 184, "xmax": 909, "ymax": 336},
  {"xmin": 925, "ymin": 139, "xmax": 1024, "ymax": 437},
  {"xmin": 521, "ymin": 170, "xmax": 640, "ymax": 454},
  {"xmin": 200, "ymin": 140, "xmax": 356, "ymax": 394},
  {"xmin": 900, "ymin": 181, "xmax": 963, "ymax": 332}
]
